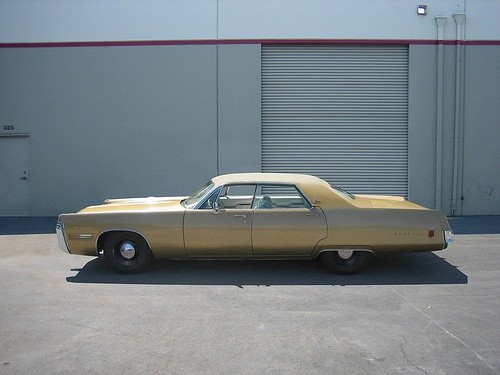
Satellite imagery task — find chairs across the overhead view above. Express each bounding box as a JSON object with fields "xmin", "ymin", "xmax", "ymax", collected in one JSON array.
[{"xmin": 258, "ymin": 195, "xmax": 272, "ymax": 208}]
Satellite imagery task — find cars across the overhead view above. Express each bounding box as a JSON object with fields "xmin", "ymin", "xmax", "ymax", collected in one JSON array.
[{"xmin": 54, "ymin": 172, "xmax": 456, "ymax": 274}]
